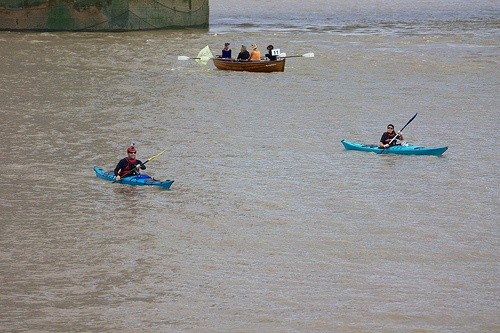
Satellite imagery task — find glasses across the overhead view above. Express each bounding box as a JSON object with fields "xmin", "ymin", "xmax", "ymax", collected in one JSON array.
[
  {"xmin": 130, "ymin": 152, "xmax": 136, "ymax": 154},
  {"xmin": 388, "ymin": 127, "xmax": 393, "ymax": 129}
]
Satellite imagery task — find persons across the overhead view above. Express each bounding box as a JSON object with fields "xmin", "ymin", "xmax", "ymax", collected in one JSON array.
[
  {"xmin": 265, "ymin": 44, "xmax": 277, "ymax": 60},
  {"xmin": 247, "ymin": 44, "xmax": 260, "ymax": 62},
  {"xmin": 114, "ymin": 145, "xmax": 146, "ymax": 180},
  {"xmin": 237, "ymin": 44, "xmax": 250, "ymax": 61},
  {"xmin": 222, "ymin": 42, "xmax": 231, "ymax": 59},
  {"xmin": 378, "ymin": 124, "xmax": 404, "ymax": 149}
]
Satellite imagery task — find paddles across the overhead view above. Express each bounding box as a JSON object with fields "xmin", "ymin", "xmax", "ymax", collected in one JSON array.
[
  {"xmin": 112, "ymin": 148, "xmax": 167, "ymax": 183},
  {"xmin": 178, "ymin": 56, "xmax": 200, "ymax": 61},
  {"xmin": 280, "ymin": 52, "xmax": 314, "ymax": 58},
  {"xmin": 201, "ymin": 56, "xmax": 219, "ymax": 62},
  {"xmin": 380, "ymin": 112, "xmax": 418, "ymax": 154}
]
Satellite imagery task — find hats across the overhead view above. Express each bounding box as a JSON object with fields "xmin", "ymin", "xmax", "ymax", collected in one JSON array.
[{"xmin": 127, "ymin": 146, "xmax": 136, "ymax": 153}]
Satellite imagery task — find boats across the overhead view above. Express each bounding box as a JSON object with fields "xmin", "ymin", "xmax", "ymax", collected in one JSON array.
[
  {"xmin": 341, "ymin": 139, "xmax": 448, "ymax": 157},
  {"xmin": 213, "ymin": 58, "xmax": 285, "ymax": 72},
  {"xmin": 92, "ymin": 164, "xmax": 175, "ymax": 191}
]
